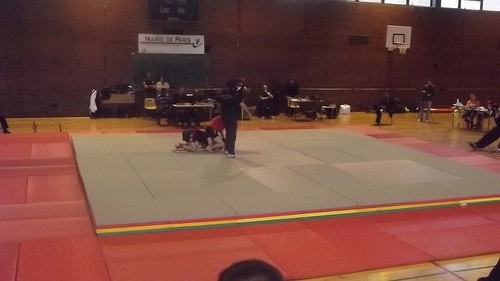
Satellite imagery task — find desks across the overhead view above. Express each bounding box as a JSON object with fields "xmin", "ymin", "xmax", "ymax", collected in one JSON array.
[
  {"xmin": 452, "ymin": 109, "xmax": 491, "ymax": 132},
  {"xmin": 172, "ymin": 103, "xmax": 213, "ymax": 125},
  {"xmin": 290, "ymin": 99, "xmax": 324, "ymax": 120}
]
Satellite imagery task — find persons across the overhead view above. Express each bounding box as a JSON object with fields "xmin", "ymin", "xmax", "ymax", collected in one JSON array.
[{"xmin": 143, "ymin": 71, "xmax": 500, "ymax": 159}]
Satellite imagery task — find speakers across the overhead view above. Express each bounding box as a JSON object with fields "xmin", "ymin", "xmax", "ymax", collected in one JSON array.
[{"xmin": 350, "ymin": 35, "xmax": 369, "ymax": 44}]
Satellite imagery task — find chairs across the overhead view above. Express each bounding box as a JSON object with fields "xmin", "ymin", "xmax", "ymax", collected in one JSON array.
[
  {"xmin": 288, "ymin": 98, "xmax": 300, "ymax": 117},
  {"xmin": 144, "ymin": 98, "xmax": 158, "ymax": 118}
]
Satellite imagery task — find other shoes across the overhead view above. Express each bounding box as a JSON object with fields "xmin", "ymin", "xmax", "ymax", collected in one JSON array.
[
  {"xmin": 228, "ymin": 154, "xmax": 234, "ymax": 158},
  {"xmin": 381, "ymin": 109, "xmax": 386, "ymax": 113},
  {"xmin": 226, "ymin": 150, "xmax": 228, "ymax": 154},
  {"xmin": 468, "ymin": 141, "xmax": 477, "ymax": 149},
  {"xmin": 374, "ymin": 122, "xmax": 379, "ymax": 126}
]
